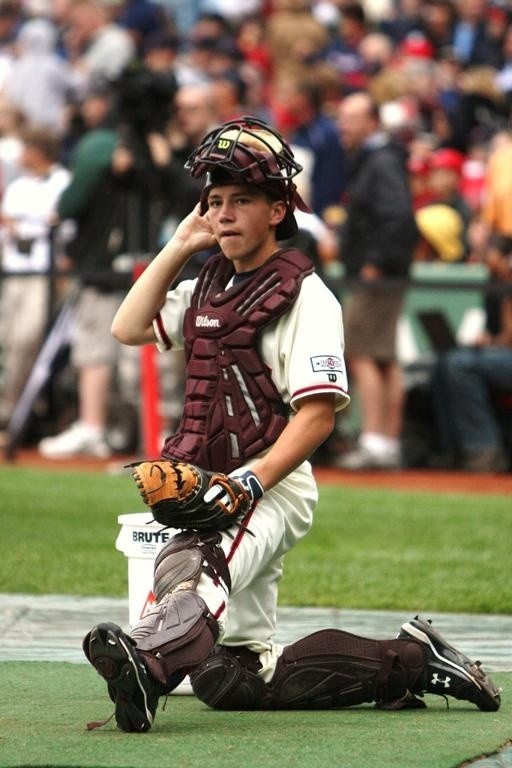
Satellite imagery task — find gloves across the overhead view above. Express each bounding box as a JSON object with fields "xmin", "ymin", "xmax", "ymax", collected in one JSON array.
[{"xmin": 204, "ymin": 467, "xmax": 264, "ymax": 519}]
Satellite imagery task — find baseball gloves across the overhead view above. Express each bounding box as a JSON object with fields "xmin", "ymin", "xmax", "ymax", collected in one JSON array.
[{"xmin": 123, "ymin": 457, "xmax": 263, "ymax": 531}]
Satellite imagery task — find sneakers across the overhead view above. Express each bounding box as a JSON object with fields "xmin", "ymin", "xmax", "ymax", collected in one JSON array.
[
  {"xmin": 82, "ymin": 622, "xmax": 159, "ymax": 733},
  {"xmin": 37, "ymin": 421, "xmax": 113, "ymax": 459},
  {"xmin": 396, "ymin": 615, "xmax": 500, "ymax": 711}
]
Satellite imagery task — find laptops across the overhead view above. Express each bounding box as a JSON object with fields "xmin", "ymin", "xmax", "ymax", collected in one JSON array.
[{"xmin": 417, "ymin": 309, "xmax": 481, "ymax": 353}]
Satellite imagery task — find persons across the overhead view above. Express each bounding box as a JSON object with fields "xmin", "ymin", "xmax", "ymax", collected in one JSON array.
[
  {"xmin": 1, "ymin": 1, "xmax": 511, "ymax": 474},
  {"xmin": 82, "ymin": 114, "xmax": 502, "ymax": 733}
]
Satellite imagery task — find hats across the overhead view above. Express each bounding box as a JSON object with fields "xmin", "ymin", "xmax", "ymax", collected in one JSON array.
[{"xmin": 416, "ymin": 205, "xmax": 464, "ymax": 261}]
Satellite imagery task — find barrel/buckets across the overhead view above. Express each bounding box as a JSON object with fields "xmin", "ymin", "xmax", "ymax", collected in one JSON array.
[{"xmin": 110, "ymin": 508, "xmax": 197, "ymax": 697}]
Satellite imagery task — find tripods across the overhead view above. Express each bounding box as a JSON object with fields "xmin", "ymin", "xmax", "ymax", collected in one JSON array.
[{"xmin": 4, "ymin": 136, "xmax": 191, "ymax": 464}]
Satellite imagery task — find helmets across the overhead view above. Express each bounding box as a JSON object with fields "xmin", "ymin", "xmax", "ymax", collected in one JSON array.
[{"xmin": 183, "ymin": 117, "xmax": 298, "ymax": 241}]
